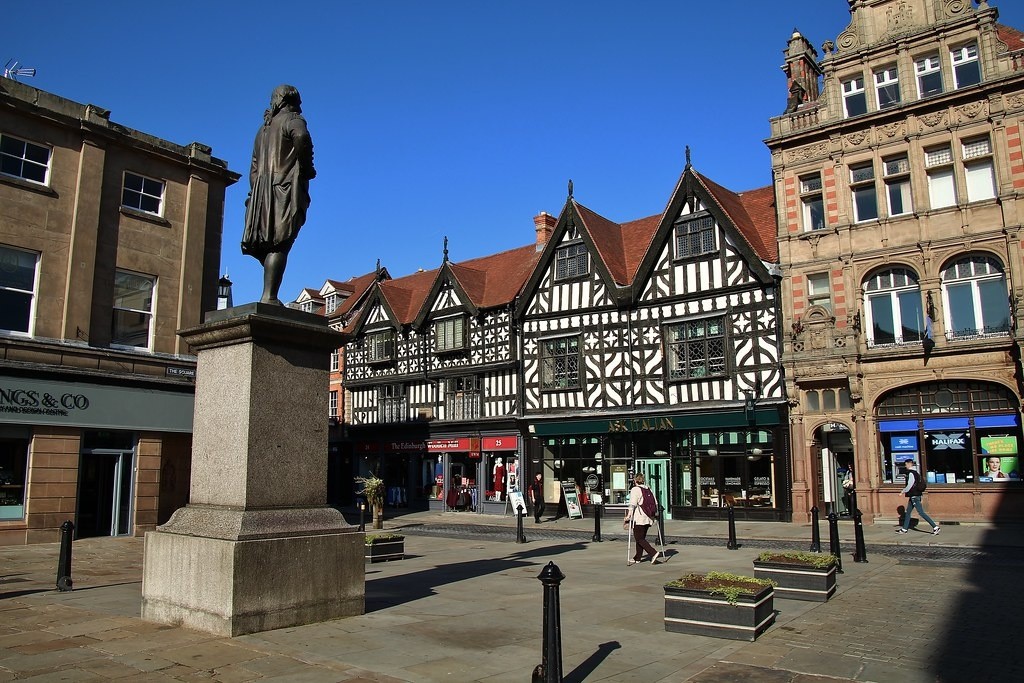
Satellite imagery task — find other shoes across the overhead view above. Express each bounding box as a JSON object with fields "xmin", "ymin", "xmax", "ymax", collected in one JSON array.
[
  {"xmin": 630, "ymin": 559, "xmax": 641, "ymax": 564},
  {"xmin": 651, "ymin": 552, "xmax": 660, "ymax": 564},
  {"xmin": 931, "ymin": 526, "xmax": 941, "ymax": 536},
  {"xmin": 894, "ymin": 528, "xmax": 908, "ymax": 534}
]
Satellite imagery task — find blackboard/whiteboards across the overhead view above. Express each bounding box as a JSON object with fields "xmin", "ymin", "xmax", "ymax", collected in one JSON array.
[
  {"xmin": 508, "ymin": 492, "xmax": 528, "ymax": 516},
  {"xmin": 565, "ymin": 492, "xmax": 582, "ymax": 517}
]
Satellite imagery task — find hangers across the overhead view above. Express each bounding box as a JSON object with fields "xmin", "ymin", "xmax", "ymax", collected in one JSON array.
[{"xmin": 451, "ymin": 483, "xmax": 475, "ymax": 495}]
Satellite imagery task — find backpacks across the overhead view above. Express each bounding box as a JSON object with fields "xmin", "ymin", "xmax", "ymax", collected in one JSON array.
[
  {"xmin": 911, "ymin": 470, "xmax": 926, "ymax": 492},
  {"xmin": 635, "ymin": 485, "xmax": 657, "ymax": 519}
]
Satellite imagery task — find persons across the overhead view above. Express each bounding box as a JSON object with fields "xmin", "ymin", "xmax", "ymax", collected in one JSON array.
[
  {"xmin": 492, "ymin": 457, "xmax": 505, "ymax": 500},
  {"xmin": 984, "ymin": 456, "xmax": 1009, "ymax": 478},
  {"xmin": 844, "ymin": 461, "xmax": 854, "ymax": 516},
  {"xmin": 895, "ymin": 459, "xmax": 940, "ymax": 536},
  {"xmin": 532, "ymin": 473, "xmax": 545, "ymax": 524},
  {"xmin": 624, "ymin": 473, "xmax": 659, "ymax": 564},
  {"xmin": 434, "ymin": 457, "xmax": 443, "ymax": 481},
  {"xmin": 510, "ymin": 475, "xmax": 515, "ymax": 486},
  {"xmin": 241, "ymin": 85, "xmax": 316, "ymax": 305}
]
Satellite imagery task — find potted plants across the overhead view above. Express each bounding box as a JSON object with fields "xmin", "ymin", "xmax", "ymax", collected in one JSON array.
[
  {"xmin": 364, "ymin": 533, "xmax": 405, "ymax": 564},
  {"xmin": 663, "ymin": 573, "xmax": 776, "ymax": 641},
  {"xmin": 752, "ymin": 550, "xmax": 838, "ymax": 603}
]
